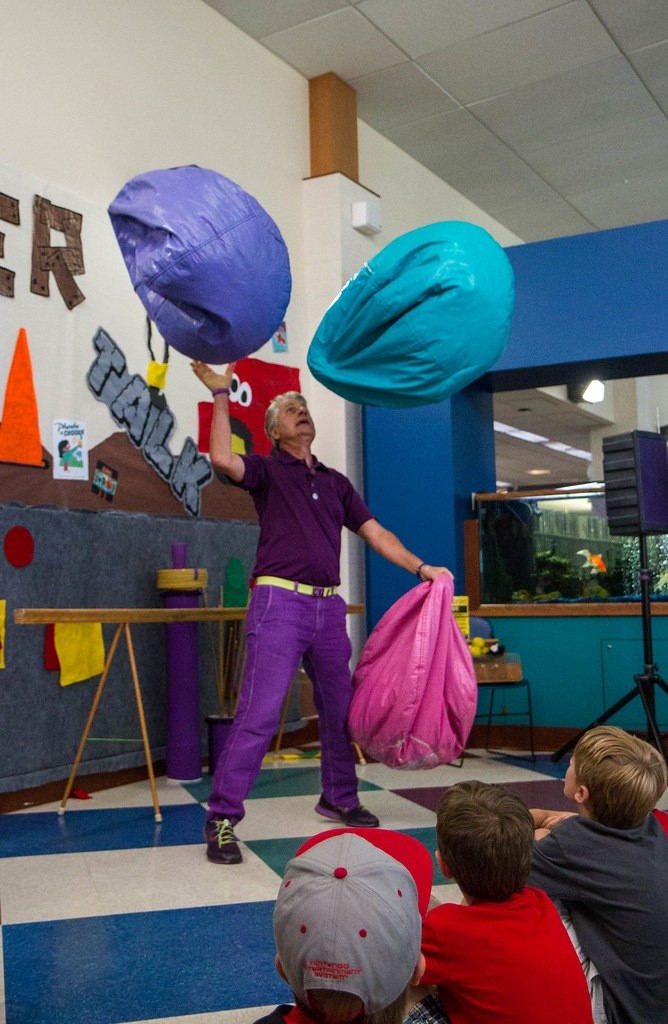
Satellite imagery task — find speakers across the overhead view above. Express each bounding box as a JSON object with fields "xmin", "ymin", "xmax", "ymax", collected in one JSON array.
[{"xmin": 602, "ymin": 429, "xmax": 668, "ymax": 539}]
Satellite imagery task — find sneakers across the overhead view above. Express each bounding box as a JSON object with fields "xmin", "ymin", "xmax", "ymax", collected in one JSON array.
[
  {"xmin": 314, "ymin": 792, "xmax": 379, "ymax": 828},
  {"xmin": 204, "ymin": 818, "xmax": 242, "ymax": 864}
]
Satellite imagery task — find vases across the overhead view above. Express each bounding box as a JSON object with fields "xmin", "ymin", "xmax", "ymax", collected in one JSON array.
[{"xmin": 203, "ymin": 714, "xmax": 236, "ymax": 778}]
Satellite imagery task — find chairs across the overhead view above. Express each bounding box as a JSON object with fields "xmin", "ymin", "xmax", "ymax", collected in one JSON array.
[{"xmin": 438, "ymin": 616, "xmax": 539, "ymax": 768}]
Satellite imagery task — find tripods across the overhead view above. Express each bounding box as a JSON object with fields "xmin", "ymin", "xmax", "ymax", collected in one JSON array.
[{"xmin": 552, "ymin": 536, "xmax": 668, "ymax": 772}]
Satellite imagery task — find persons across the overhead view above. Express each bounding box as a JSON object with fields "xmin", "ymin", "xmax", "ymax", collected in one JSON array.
[
  {"xmin": 526, "ymin": 725, "xmax": 667, "ymax": 1024},
  {"xmin": 191, "ymin": 356, "xmax": 455, "ymax": 865},
  {"xmin": 396, "ymin": 780, "xmax": 595, "ymax": 1024},
  {"xmin": 253, "ymin": 827, "xmax": 433, "ymax": 1024}
]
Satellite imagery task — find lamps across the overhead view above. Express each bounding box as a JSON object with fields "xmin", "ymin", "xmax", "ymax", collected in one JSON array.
[{"xmin": 566, "ymin": 379, "xmax": 605, "ymax": 404}]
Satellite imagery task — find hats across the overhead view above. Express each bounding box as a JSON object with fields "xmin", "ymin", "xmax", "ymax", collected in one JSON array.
[{"xmin": 275, "ymin": 830, "xmax": 433, "ymax": 1014}]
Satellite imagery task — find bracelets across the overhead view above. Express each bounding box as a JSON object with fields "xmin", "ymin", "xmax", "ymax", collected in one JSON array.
[
  {"xmin": 417, "ymin": 563, "xmax": 426, "ymax": 580},
  {"xmin": 211, "ymin": 388, "xmax": 230, "ymax": 397}
]
